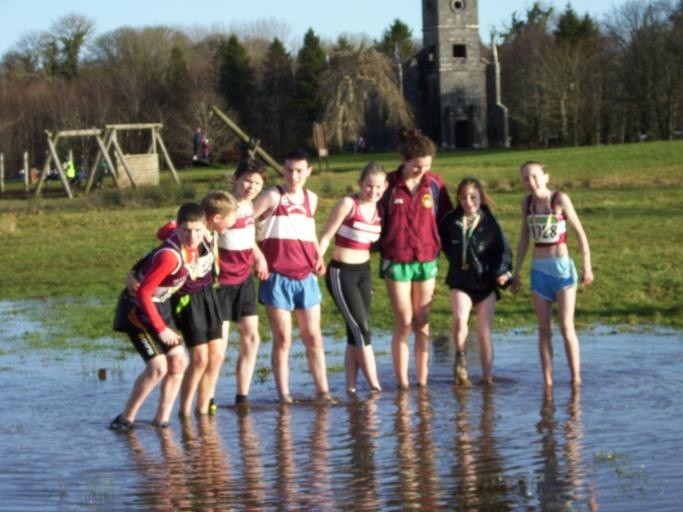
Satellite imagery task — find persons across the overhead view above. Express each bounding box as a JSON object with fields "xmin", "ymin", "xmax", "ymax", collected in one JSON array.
[
  {"xmin": 438, "ymin": 178, "xmax": 513, "ymax": 386},
  {"xmin": 29, "ymin": 157, "xmax": 87, "ymax": 187},
  {"xmin": 253, "ymin": 149, "xmax": 335, "ymax": 403},
  {"xmin": 372, "ymin": 129, "xmax": 455, "ymax": 390},
  {"xmin": 157, "ymin": 158, "xmax": 269, "ymax": 412},
  {"xmin": 200, "ymin": 136, "xmax": 209, "ymax": 160},
  {"xmin": 125, "ymin": 189, "xmax": 239, "ymax": 418},
  {"xmin": 109, "ymin": 203, "xmax": 207, "ymax": 431},
  {"xmin": 511, "ymin": 161, "xmax": 594, "ymax": 385},
  {"xmin": 319, "ymin": 161, "xmax": 389, "ymax": 393},
  {"xmin": 191, "ymin": 126, "xmax": 203, "ymax": 160}
]
